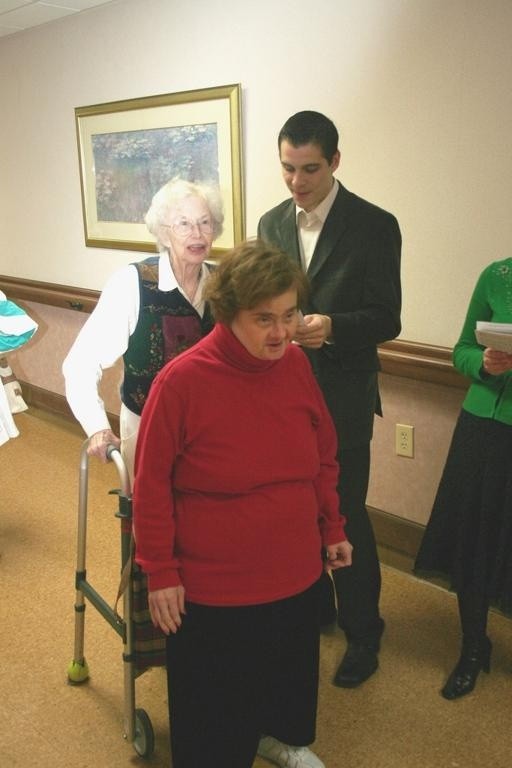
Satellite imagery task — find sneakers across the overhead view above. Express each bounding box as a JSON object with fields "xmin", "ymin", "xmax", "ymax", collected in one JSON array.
[{"xmin": 257, "ymin": 732, "xmax": 325, "ymax": 768}]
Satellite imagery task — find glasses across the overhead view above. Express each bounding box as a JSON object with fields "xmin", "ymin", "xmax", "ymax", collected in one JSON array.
[{"xmin": 157, "ymin": 218, "xmax": 220, "ymax": 237}]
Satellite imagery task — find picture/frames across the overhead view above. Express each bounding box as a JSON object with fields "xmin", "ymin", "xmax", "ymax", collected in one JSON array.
[{"xmin": 71, "ymin": 82, "xmax": 249, "ymax": 262}]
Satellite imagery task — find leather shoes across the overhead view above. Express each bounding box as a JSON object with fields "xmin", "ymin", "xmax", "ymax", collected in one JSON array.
[{"xmin": 334, "ymin": 618, "xmax": 385, "ymax": 689}]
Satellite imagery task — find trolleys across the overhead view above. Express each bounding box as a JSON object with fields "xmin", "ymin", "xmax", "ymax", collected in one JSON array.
[{"xmin": 69, "ymin": 437, "xmax": 168, "ymax": 757}]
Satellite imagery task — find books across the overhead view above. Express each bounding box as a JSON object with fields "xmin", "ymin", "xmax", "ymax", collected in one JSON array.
[{"xmin": 472, "ymin": 316, "xmax": 511, "ymax": 359}]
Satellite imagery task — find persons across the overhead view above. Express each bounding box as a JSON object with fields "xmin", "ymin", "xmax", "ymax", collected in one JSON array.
[
  {"xmin": 59, "ymin": 172, "xmax": 220, "ymax": 685},
  {"xmin": 254, "ymin": 107, "xmax": 404, "ymax": 694},
  {"xmin": 126, "ymin": 238, "xmax": 355, "ymax": 768},
  {"xmin": 412, "ymin": 255, "xmax": 512, "ymax": 702}
]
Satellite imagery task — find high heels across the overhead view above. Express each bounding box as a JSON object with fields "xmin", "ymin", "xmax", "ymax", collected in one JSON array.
[{"xmin": 442, "ymin": 637, "xmax": 493, "ymax": 699}]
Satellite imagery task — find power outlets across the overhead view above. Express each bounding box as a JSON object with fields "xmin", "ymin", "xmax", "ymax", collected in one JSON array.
[{"xmin": 395, "ymin": 423, "xmax": 415, "ymax": 458}]
